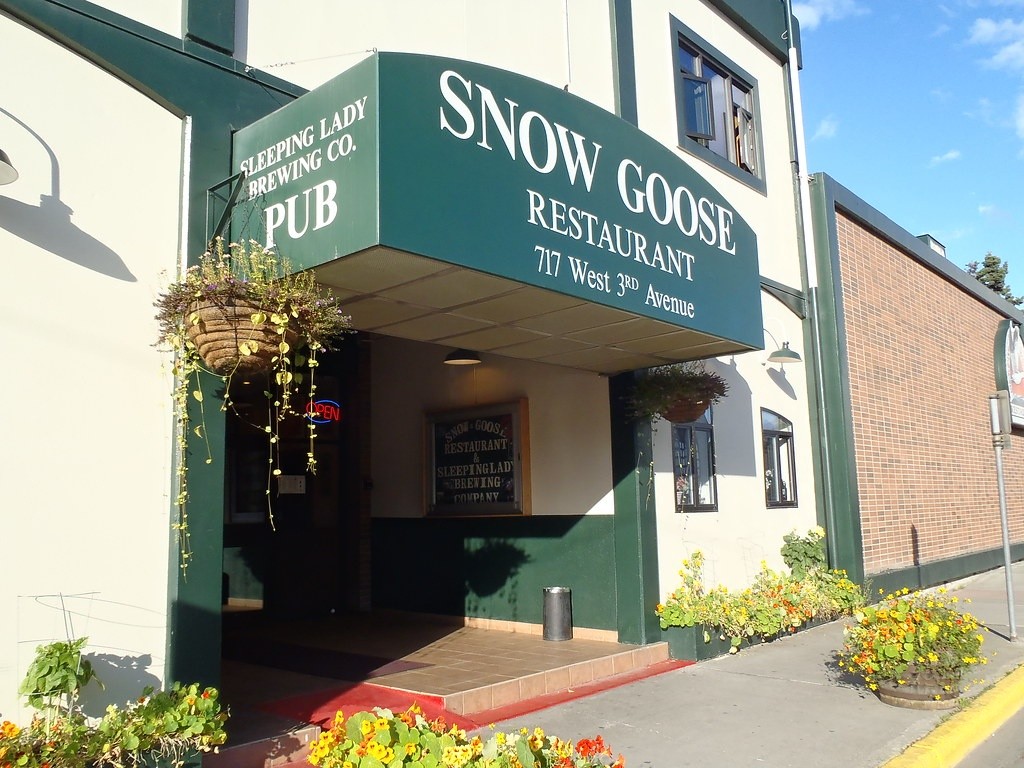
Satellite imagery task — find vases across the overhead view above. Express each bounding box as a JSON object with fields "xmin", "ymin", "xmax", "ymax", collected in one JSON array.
[
  {"xmin": 184, "ymin": 293, "xmax": 304, "ymax": 378},
  {"xmin": 656, "ymin": 392, "xmax": 712, "ymax": 424},
  {"xmin": 879, "ymin": 664, "xmax": 959, "ymax": 710}
]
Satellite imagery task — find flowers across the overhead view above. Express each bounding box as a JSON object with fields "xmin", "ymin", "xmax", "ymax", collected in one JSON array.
[
  {"xmin": 150, "ymin": 237, "xmax": 358, "ymax": 587},
  {"xmin": 0, "ymin": 637, "xmax": 627, "ymax": 768},
  {"xmin": 837, "ymin": 586, "xmax": 988, "ymax": 699},
  {"xmin": 654, "ymin": 524, "xmax": 864, "ymax": 654},
  {"xmin": 624, "ymin": 362, "xmax": 731, "ymax": 529}
]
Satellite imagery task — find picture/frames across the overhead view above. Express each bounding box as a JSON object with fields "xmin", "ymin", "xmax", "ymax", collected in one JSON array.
[{"xmin": 422, "ymin": 399, "xmax": 531, "ymax": 517}]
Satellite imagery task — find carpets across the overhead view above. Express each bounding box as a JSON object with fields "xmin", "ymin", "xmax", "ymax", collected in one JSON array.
[{"xmin": 222, "ymin": 630, "xmax": 435, "ymax": 682}]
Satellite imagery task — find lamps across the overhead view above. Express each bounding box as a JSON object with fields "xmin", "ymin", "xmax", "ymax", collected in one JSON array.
[
  {"xmin": 767, "ymin": 340, "xmax": 803, "ymax": 363},
  {"xmin": 443, "ymin": 349, "xmax": 482, "ymax": 365},
  {"xmin": 0, "ymin": 149, "xmax": 18, "ymax": 186}
]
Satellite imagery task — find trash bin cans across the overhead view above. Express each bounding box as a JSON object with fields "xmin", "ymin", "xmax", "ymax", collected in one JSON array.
[{"xmin": 542, "ymin": 587, "xmax": 573, "ymax": 642}]
